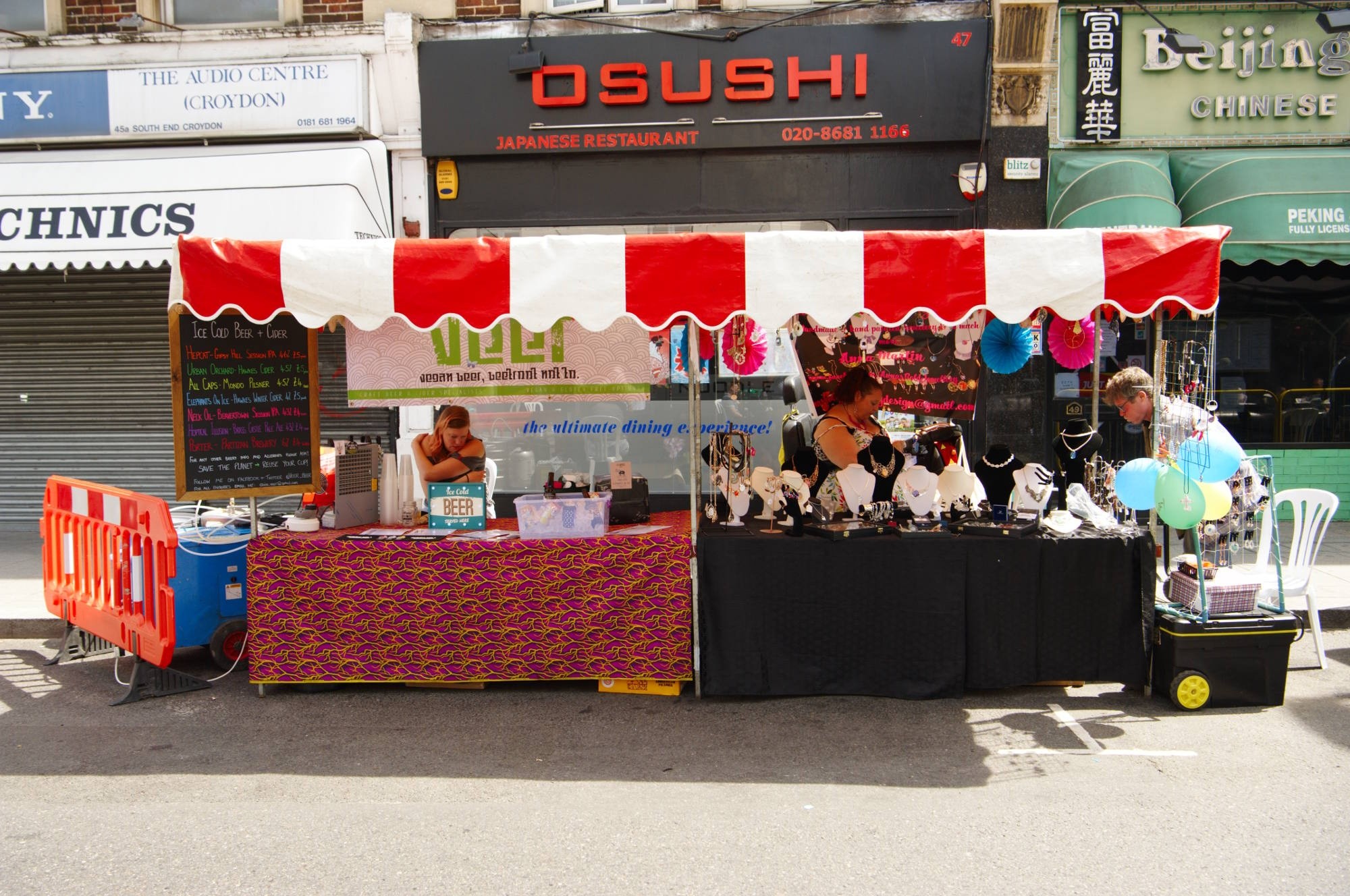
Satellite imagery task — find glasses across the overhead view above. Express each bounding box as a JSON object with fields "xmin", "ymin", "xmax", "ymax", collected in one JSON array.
[{"xmin": 1118, "ymin": 390, "xmax": 1147, "ymax": 412}]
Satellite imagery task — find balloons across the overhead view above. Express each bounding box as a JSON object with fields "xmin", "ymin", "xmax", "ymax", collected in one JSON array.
[{"xmin": 1113, "ymin": 430, "xmax": 1242, "ymax": 530}]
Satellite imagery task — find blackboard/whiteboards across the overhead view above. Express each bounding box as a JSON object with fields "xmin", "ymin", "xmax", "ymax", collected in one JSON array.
[{"xmin": 169, "ymin": 304, "xmax": 321, "ymax": 502}]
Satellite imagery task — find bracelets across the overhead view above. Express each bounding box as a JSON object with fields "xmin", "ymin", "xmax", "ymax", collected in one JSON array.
[{"xmin": 466, "ymin": 474, "xmax": 469, "ymax": 483}]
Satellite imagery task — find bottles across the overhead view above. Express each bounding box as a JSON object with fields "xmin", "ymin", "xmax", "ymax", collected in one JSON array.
[
  {"xmin": 582, "ymin": 489, "xmax": 603, "ymax": 528},
  {"xmin": 542, "ymin": 472, "xmax": 556, "ymax": 501}
]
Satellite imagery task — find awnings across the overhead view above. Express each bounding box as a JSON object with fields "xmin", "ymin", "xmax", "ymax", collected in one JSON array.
[{"xmin": 1048, "ymin": 143, "xmax": 1349, "ymax": 267}]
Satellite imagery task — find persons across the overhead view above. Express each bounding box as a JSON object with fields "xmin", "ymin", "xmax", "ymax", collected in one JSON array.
[
  {"xmin": 411, "ymin": 406, "xmax": 486, "ymax": 503},
  {"xmin": 1102, "ymin": 365, "xmax": 1268, "ymax": 566},
  {"xmin": 723, "ymin": 383, "xmax": 749, "ymax": 419},
  {"xmin": 812, "ymin": 367, "xmax": 891, "ymax": 493}
]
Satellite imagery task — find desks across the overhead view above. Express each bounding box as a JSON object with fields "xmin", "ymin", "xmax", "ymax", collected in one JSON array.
[
  {"xmin": 696, "ymin": 514, "xmax": 1142, "ymax": 687},
  {"xmin": 247, "ymin": 513, "xmax": 694, "ymax": 698}
]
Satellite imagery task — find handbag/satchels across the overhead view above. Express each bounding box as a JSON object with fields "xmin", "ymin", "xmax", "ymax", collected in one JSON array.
[{"xmin": 595, "ymin": 475, "xmax": 649, "ymax": 524}]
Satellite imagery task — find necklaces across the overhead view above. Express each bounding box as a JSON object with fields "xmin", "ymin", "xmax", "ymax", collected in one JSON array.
[
  {"xmin": 1023, "ymin": 469, "xmax": 1046, "ymax": 502},
  {"xmin": 983, "ymin": 453, "xmax": 1014, "ymax": 468},
  {"xmin": 843, "ymin": 469, "xmax": 869, "ymax": 503},
  {"xmin": 906, "ymin": 471, "xmax": 930, "ymax": 497},
  {"xmin": 706, "ymin": 429, "xmax": 804, "ymax": 522},
  {"xmin": 1160, "ymin": 340, "xmax": 1206, "ymax": 460},
  {"xmin": 927, "ymin": 339, "xmax": 947, "ymax": 361},
  {"xmin": 968, "ymin": 311, "xmax": 986, "ymax": 360},
  {"xmin": 868, "ymin": 444, "xmax": 895, "ymax": 477},
  {"xmin": 1061, "ymin": 425, "xmax": 1092, "ymax": 459},
  {"xmin": 871, "ymin": 501, "xmax": 894, "ymax": 522},
  {"xmin": 943, "ymin": 472, "xmax": 974, "ymax": 499}
]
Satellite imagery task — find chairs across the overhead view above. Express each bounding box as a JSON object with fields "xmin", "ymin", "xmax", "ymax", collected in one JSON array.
[
  {"xmin": 1283, "ymin": 408, "xmax": 1320, "ymax": 443},
  {"xmin": 1251, "ymin": 488, "xmax": 1339, "ymax": 670}
]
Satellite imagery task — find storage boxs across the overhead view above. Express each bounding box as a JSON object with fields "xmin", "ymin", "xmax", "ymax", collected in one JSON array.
[
  {"xmin": 1146, "ymin": 599, "xmax": 1290, "ymax": 719},
  {"xmin": 513, "ymin": 492, "xmax": 613, "ymax": 540},
  {"xmin": 598, "ymin": 679, "xmax": 684, "ymax": 693}
]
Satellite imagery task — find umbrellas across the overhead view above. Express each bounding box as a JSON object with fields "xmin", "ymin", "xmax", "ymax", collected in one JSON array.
[
  {"xmin": 722, "ymin": 317, "xmax": 767, "ymax": 376},
  {"xmin": 980, "ymin": 320, "xmax": 1032, "ymax": 373},
  {"xmin": 679, "ymin": 323, "xmax": 706, "ymax": 372},
  {"xmin": 1047, "ymin": 317, "xmax": 1103, "ymax": 370}
]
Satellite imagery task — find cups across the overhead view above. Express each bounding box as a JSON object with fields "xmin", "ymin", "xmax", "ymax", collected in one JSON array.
[{"xmin": 380, "ymin": 452, "xmax": 414, "ymax": 523}]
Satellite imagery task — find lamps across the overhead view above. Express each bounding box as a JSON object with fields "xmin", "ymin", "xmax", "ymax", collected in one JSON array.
[
  {"xmin": 1135, "ymin": 0, "xmax": 1205, "ymax": 54},
  {"xmin": 1296, "ymin": 0, "xmax": 1350, "ymax": 34},
  {"xmin": 509, "ymin": 11, "xmax": 543, "ymax": 77},
  {"xmin": 114, "ymin": 12, "xmax": 186, "ymax": 34}
]
